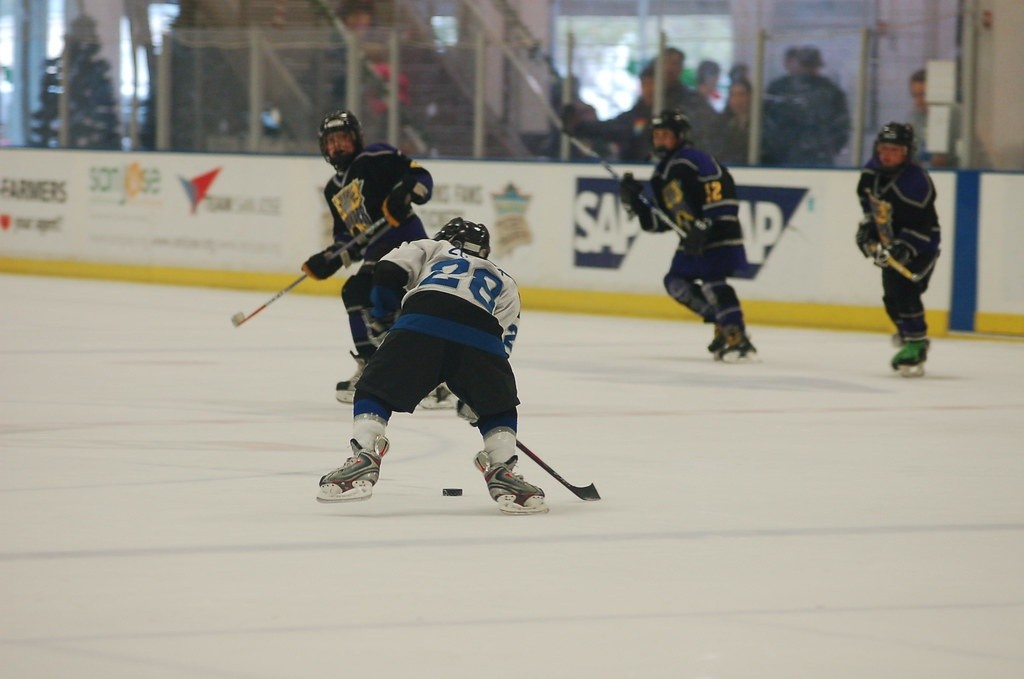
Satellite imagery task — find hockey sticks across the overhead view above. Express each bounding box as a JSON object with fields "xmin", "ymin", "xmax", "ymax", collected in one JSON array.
[
  {"xmin": 568, "ymin": 136, "xmax": 689, "ymax": 240},
  {"xmin": 229, "ymin": 216, "xmax": 388, "ymax": 329},
  {"xmin": 869, "ymin": 243, "xmax": 942, "ymax": 283},
  {"xmin": 361, "ymin": 309, "xmax": 602, "ymax": 502}
]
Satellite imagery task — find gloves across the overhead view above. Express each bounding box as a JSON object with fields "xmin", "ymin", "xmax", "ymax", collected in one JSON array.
[
  {"xmin": 301, "ymin": 247, "xmax": 344, "ymax": 281},
  {"xmin": 382, "ymin": 187, "xmax": 412, "ymax": 226},
  {"xmin": 856, "ymin": 220, "xmax": 880, "ymax": 255},
  {"xmin": 874, "ymin": 241, "xmax": 912, "ymax": 268}
]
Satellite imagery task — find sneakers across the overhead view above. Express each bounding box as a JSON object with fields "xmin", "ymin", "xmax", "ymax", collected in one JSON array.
[
  {"xmin": 336, "ymin": 348, "xmax": 369, "ymax": 401},
  {"xmin": 891, "ymin": 329, "xmax": 930, "ymax": 379},
  {"xmin": 704, "ymin": 308, "xmax": 757, "ymax": 361},
  {"xmin": 474, "ymin": 450, "xmax": 549, "ymax": 514},
  {"xmin": 316, "ymin": 433, "xmax": 389, "ymax": 500}
]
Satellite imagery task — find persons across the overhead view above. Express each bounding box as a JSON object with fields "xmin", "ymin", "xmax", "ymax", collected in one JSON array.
[
  {"xmin": 544, "ymin": 49, "xmax": 753, "ymax": 164},
  {"xmin": 620, "ymin": 106, "xmax": 757, "ymax": 358},
  {"xmin": 41, "ymin": 17, "xmax": 121, "ymax": 149},
  {"xmin": 857, "ymin": 121, "xmax": 941, "ymax": 369},
  {"xmin": 310, "ymin": 0, "xmax": 449, "ymax": 149},
  {"xmin": 900, "ymin": 69, "xmax": 962, "ymax": 168},
  {"xmin": 139, "ymin": 17, "xmax": 240, "ymax": 151},
  {"xmin": 761, "ymin": 47, "xmax": 850, "ymax": 164},
  {"xmin": 300, "ymin": 108, "xmax": 451, "ymax": 403},
  {"xmin": 320, "ymin": 216, "xmax": 544, "ymax": 504}
]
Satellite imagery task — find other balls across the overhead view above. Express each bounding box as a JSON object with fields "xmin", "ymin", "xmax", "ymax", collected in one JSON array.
[{"xmin": 442, "ymin": 488, "xmax": 463, "ymax": 497}]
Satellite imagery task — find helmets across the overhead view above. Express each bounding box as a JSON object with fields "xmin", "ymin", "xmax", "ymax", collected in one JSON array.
[
  {"xmin": 648, "ymin": 110, "xmax": 692, "ymax": 133},
  {"xmin": 434, "ymin": 218, "xmax": 491, "ymax": 260},
  {"xmin": 318, "ymin": 109, "xmax": 363, "ymax": 161},
  {"xmin": 874, "ymin": 123, "xmax": 914, "ymax": 155}
]
